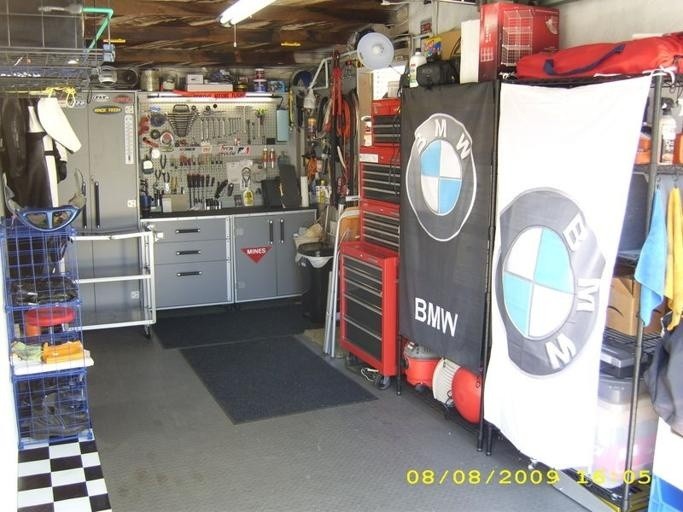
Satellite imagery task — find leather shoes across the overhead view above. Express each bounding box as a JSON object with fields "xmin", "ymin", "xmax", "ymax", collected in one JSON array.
[{"xmin": 21, "ymin": 391, "xmax": 86, "ymax": 439}]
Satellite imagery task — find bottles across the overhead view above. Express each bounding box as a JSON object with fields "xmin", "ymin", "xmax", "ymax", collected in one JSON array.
[
  {"xmin": 408, "ymin": 48, "xmax": 427, "ymax": 89},
  {"xmin": 657, "ymin": 98, "xmax": 677, "ymax": 166}
]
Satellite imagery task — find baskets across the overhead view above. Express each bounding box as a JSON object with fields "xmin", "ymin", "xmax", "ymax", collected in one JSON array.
[
  {"xmin": 10, "ymin": 373, "xmax": 93, "ymax": 448},
  {"xmin": 0, "ymin": 218, "xmax": 88, "ymax": 378}
]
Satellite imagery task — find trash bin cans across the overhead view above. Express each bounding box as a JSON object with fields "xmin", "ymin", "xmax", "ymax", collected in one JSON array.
[{"xmin": 298, "ymin": 242, "xmax": 338, "ymax": 323}]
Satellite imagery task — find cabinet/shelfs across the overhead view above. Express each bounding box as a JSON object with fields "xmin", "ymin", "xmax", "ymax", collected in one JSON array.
[
  {"xmin": 44, "ymin": 89, "xmax": 141, "ymax": 332},
  {"xmin": 398, "ymin": 82, "xmax": 496, "ymax": 450},
  {"xmin": 231, "ymin": 210, "xmax": 315, "ymax": 303},
  {"xmin": 138, "ymin": 96, "xmax": 300, "ymax": 213},
  {"xmin": 143, "ymin": 216, "xmax": 235, "ymax": 309},
  {"xmin": 489, "ymin": 72, "xmax": 682, "ymax": 510}
]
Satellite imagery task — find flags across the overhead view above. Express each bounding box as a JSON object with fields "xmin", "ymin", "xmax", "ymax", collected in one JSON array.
[
  {"xmin": 481, "ymin": 75, "xmax": 654, "ymax": 470},
  {"xmin": 398, "ymin": 79, "xmax": 498, "ymax": 378}
]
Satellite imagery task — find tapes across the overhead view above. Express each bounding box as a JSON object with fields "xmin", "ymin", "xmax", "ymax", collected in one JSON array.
[{"xmin": 151, "ymin": 129, "xmax": 173, "ymax": 147}]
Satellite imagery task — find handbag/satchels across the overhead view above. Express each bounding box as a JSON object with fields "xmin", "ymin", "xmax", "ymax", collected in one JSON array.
[{"xmin": 515, "ymin": 32, "xmax": 682, "ymax": 81}]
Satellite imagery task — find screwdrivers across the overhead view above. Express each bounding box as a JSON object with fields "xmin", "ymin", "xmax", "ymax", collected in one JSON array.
[{"xmin": 180, "ymin": 154, "xmax": 223, "ymax": 208}]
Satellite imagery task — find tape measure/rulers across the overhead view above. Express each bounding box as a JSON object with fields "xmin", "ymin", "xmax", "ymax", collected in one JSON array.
[{"xmin": 255, "ymin": 108, "xmax": 265, "ymax": 137}]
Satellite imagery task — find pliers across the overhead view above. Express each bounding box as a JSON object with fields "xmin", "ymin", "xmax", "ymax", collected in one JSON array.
[
  {"xmin": 330, "ymin": 50, "xmax": 342, "ymax": 116},
  {"xmin": 154, "ymin": 154, "xmax": 177, "ymax": 194}
]
